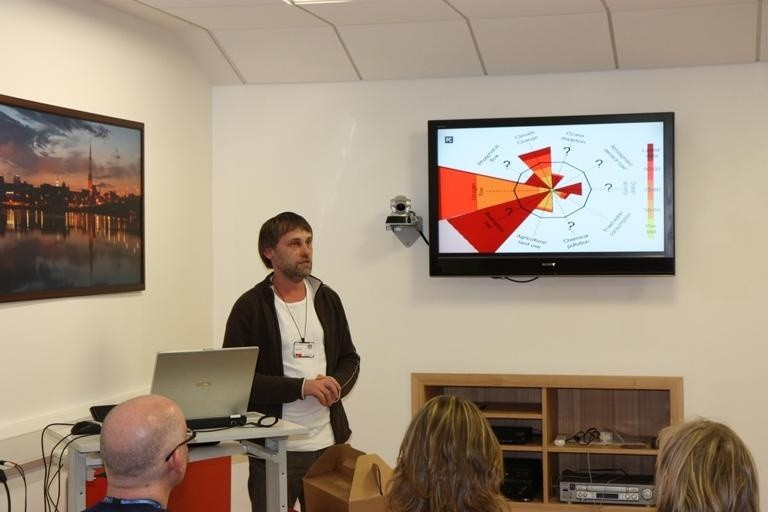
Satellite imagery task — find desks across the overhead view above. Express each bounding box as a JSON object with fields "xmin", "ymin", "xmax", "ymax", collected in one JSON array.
[{"xmin": 50, "ymin": 415, "xmax": 307, "ymax": 511}]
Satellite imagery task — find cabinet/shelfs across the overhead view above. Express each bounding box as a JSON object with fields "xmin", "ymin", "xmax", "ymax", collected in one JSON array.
[{"xmin": 409, "ymin": 372, "xmax": 685, "ymax": 512}]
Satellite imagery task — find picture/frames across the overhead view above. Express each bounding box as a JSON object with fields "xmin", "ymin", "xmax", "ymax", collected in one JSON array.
[{"xmin": 2, "ymin": 92, "xmax": 147, "ymax": 308}]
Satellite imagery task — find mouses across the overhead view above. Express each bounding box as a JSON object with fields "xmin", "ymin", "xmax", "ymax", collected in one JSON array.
[{"xmin": 70, "ymin": 421, "xmax": 101, "ymax": 435}]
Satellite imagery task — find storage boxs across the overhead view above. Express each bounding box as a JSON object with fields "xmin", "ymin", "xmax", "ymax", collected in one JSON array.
[{"xmin": 302, "ymin": 442, "xmax": 398, "ymax": 511}]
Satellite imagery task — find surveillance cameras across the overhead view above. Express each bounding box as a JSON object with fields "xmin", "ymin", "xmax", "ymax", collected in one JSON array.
[{"xmin": 386, "ymin": 195, "xmax": 417, "ymax": 226}]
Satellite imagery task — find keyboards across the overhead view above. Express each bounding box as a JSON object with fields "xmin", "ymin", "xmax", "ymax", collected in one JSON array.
[{"xmin": 90, "ymin": 405, "xmax": 118, "ymax": 422}]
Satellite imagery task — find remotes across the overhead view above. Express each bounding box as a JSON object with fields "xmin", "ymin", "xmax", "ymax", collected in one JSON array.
[{"xmin": 554, "ymin": 434, "xmax": 567, "ymax": 446}]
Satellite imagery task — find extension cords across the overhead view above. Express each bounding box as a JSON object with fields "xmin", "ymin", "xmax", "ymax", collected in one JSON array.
[{"xmin": 586, "ymin": 420, "xmax": 613, "ymax": 440}]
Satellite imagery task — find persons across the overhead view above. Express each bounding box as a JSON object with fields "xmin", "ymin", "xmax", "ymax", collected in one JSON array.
[
  {"xmin": 76, "ymin": 394, "xmax": 196, "ymax": 511},
  {"xmin": 651, "ymin": 414, "xmax": 762, "ymax": 512},
  {"xmin": 216, "ymin": 210, "xmax": 363, "ymax": 512},
  {"xmin": 384, "ymin": 392, "xmax": 514, "ymax": 511}
]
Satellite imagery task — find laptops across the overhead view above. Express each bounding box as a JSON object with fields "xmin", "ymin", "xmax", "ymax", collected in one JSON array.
[{"xmin": 151, "ymin": 345, "xmax": 259, "ymax": 434}]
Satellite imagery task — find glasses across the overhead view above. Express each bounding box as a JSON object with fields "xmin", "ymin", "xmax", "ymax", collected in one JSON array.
[{"xmin": 165, "ymin": 429, "xmax": 196, "ymax": 461}]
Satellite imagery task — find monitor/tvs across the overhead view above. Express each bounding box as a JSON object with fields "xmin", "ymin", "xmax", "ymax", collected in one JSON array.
[{"xmin": 426, "ymin": 112, "xmax": 676, "ymax": 274}]
